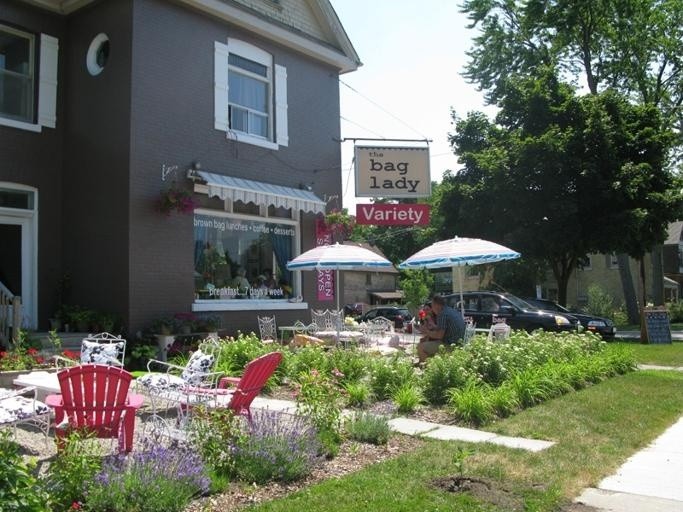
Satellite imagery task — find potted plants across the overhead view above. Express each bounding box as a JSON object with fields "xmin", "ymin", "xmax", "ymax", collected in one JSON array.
[
  {"xmin": 47, "ymin": 270, "xmax": 75, "ymax": 332},
  {"xmin": 199, "ymin": 316, "xmax": 221, "ymax": 332},
  {"xmin": 154, "ymin": 314, "xmax": 174, "ymax": 336},
  {"xmin": 66, "ymin": 305, "xmax": 95, "ymax": 332}
]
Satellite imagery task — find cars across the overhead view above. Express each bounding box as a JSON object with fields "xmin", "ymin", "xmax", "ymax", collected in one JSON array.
[
  {"xmin": 414, "ymin": 291, "xmax": 616, "ymax": 342},
  {"xmin": 345, "ymin": 303, "xmax": 369, "ymax": 317},
  {"xmin": 354, "ymin": 307, "xmax": 412, "ymax": 328}
]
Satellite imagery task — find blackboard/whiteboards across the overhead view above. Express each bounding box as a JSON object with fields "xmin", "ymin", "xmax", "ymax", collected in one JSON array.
[{"xmin": 643, "ymin": 310, "xmax": 672, "ymax": 344}]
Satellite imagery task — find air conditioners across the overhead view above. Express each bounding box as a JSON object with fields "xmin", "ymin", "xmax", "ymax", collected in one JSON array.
[{"xmin": 246, "ymin": 239, "xmax": 261, "ymax": 283}]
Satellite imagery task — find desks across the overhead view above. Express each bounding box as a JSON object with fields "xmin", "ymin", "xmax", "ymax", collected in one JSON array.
[
  {"xmin": 195, "ymin": 288, "xmax": 217, "ymax": 299},
  {"xmin": 16, "ymin": 371, "xmax": 58, "ymax": 397}
]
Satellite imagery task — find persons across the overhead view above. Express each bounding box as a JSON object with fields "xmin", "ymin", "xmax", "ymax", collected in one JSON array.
[
  {"xmin": 415, "ymin": 293, "xmax": 464, "ymax": 362},
  {"xmin": 416, "ymin": 302, "xmax": 437, "ymax": 344},
  {"xmin": 191, "ymin": 266, "xmax": 293, "ymax": 299}
]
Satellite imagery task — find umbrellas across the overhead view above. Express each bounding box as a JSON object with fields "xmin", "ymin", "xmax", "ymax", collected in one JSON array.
[
  {"xmin": 286, "ymin": 239, "xmax": 391, "ymax": 313},
  {"xmin": 397, "ymin": 234, "xmax": 521, "ymax": 319}
]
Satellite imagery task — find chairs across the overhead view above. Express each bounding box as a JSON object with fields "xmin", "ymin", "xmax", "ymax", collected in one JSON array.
[
  {"xmin": 45, "ymin": 364, "xmax": 145, "ymax": 460},
  {"xmin": 78, "ymin": 331, "xmax": 127, "ymax": 368},
  {"xmin": 480, "ymin": 296, "xmax": 500, "ymax": 313},
  {"xmin": 465, "ymin": 316, "xmax": 511, "ymax": 344},
  {"xmin": 1, "ymin": 382, "xmax": 51, "ymax": 451},
  {"xmin": 136, "ymin": 343, "xmax": 224, "ymax": 422},
  {"xmin": 254, "ymin": 311, "xmax": 391, "ymax": 360},
  {"xmin": 176, "ymin": 351, "xmax": 284, "ymax": 435}
]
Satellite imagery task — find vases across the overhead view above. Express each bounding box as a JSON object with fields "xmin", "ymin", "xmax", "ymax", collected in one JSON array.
[{"xmin": 181, "ymin": 325, "xmax": 193, "ymax": 333}]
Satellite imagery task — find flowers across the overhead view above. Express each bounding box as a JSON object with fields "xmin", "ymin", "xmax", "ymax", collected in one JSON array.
[
  {"xmin": 156, "ymin": 187, "xmax": 202, "ymax": 218},
  {"xmin": 324, "ymin": 205, "xmax": 357, "ymax": 241},
  {"xmin": 418, "ymin": 308, "xmax": 432, "ymax": 322},
  {"xmin": 175, "ymin": 313, "xmax": 199, "ymax": 322}
]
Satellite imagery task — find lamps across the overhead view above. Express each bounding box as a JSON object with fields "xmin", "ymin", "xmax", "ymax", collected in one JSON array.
[
  {"xmin": 190, "ymin": 159, "xmax": 208, "ymax": 183},
  {"xmin": 300, "ymin": 180, "xmax": 314, "ymax": 191}
]
